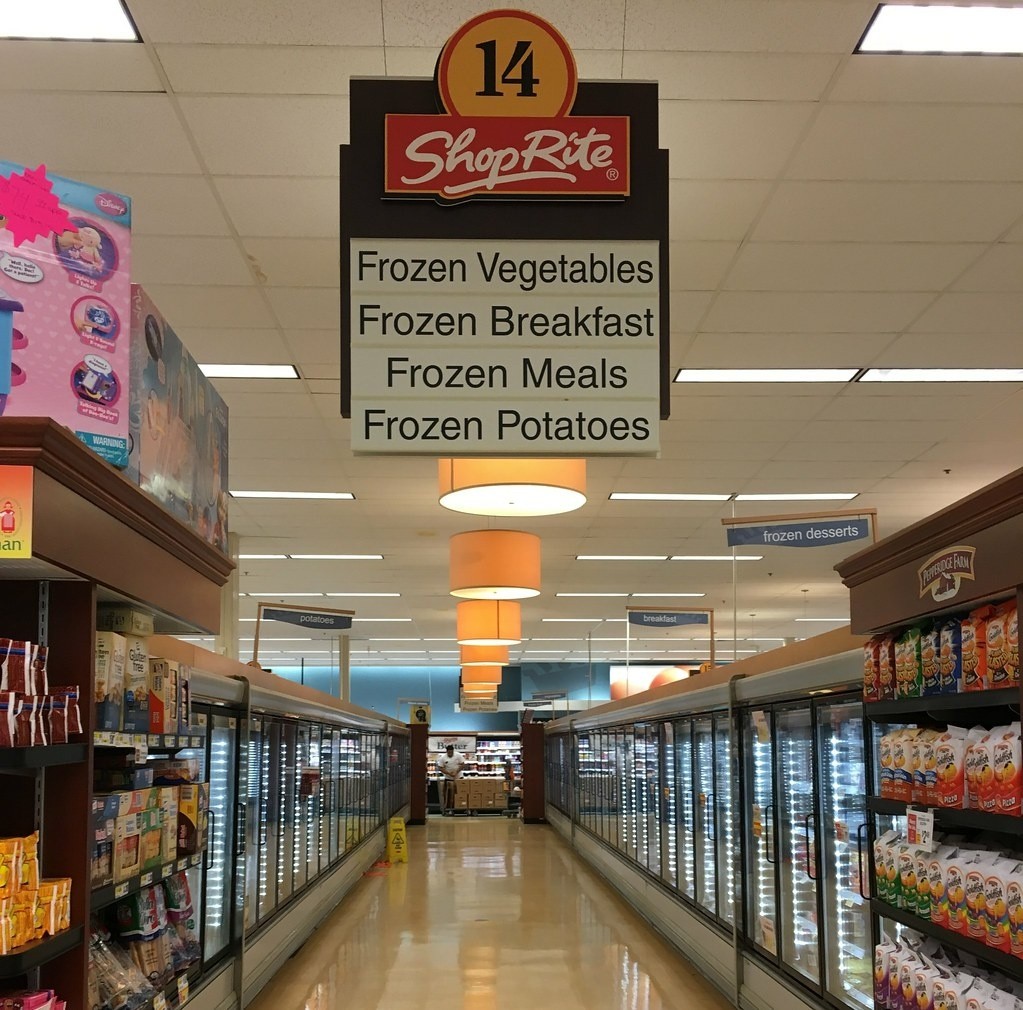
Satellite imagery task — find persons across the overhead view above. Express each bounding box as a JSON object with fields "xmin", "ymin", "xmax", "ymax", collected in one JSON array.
[{"xmin": 438, "ymin": 745, "xmax": 465, "ymax": 808}]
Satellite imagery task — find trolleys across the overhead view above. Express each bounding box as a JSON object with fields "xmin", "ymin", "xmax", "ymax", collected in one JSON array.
[
  {"xmin": 501, "ymin": 808, "xmax": 519, "ymax": 818},
  {"xmin": 442, "ymin": 778, "xmax": 508, "ymax": 816}
]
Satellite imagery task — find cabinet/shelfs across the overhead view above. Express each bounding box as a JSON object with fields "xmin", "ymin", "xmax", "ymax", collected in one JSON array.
[
  {"xmin": 428, "ymin": 731, "xmax": 476, "ymax": 779},
  {"xmin": 519, "ymin": 723, "xmax": 544, "ymax": 824},
  {"xmin": 834, "ymin": 466, "xmax": 1023, "ymax": 1010},
  {"xmin": 0, "ymin": 415, "xmax": 241, "ymax": 1010},
  {"xmin": 477, "ymin": 735, "xmax": 520, "ymax": 788},
  {"xmin": 406, "ymin": 723, "xmax": 430, "ymax": 826}
]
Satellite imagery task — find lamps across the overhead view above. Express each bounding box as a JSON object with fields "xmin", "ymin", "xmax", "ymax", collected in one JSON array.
[
  {"xmin": 437, "ymin": 458, "xmax": 587, "ymax": 517},
  {"xmin": 456, "ymin": 600, "xmax": 522, "ymax": 646},
  {"xmin": 462, "ymin": 666, "xmax": 503, "ymax": 685},
  {"xmin": 460, "ymin": 645, "xmax": 510, "ymax": 667},
  {"xmin": 464, "ymin": 685, "xmax": 497, "ymax": 693},
  {"xmin": 448, "ymin": 529, "xmax": 541, "ymax": 601}
]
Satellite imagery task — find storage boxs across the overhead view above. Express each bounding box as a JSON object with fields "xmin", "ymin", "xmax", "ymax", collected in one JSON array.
[
  {"xmin": 0, "ymin": 156, "xmax": 131, "ymax": 471},
  {"xmin": 122, "ymin": 282, "xmax": 230, "ymax": 556},
  {"xmin": 92, "ymin": 608, "xmax": 211, "ymax": 884},
  {"xmin": 454, "ymin": 779, "xmax": 508, "ymax": 808},
  {"xmin": 410, "ymin": 705, "xmax": 432, "ymax": 726}
]
